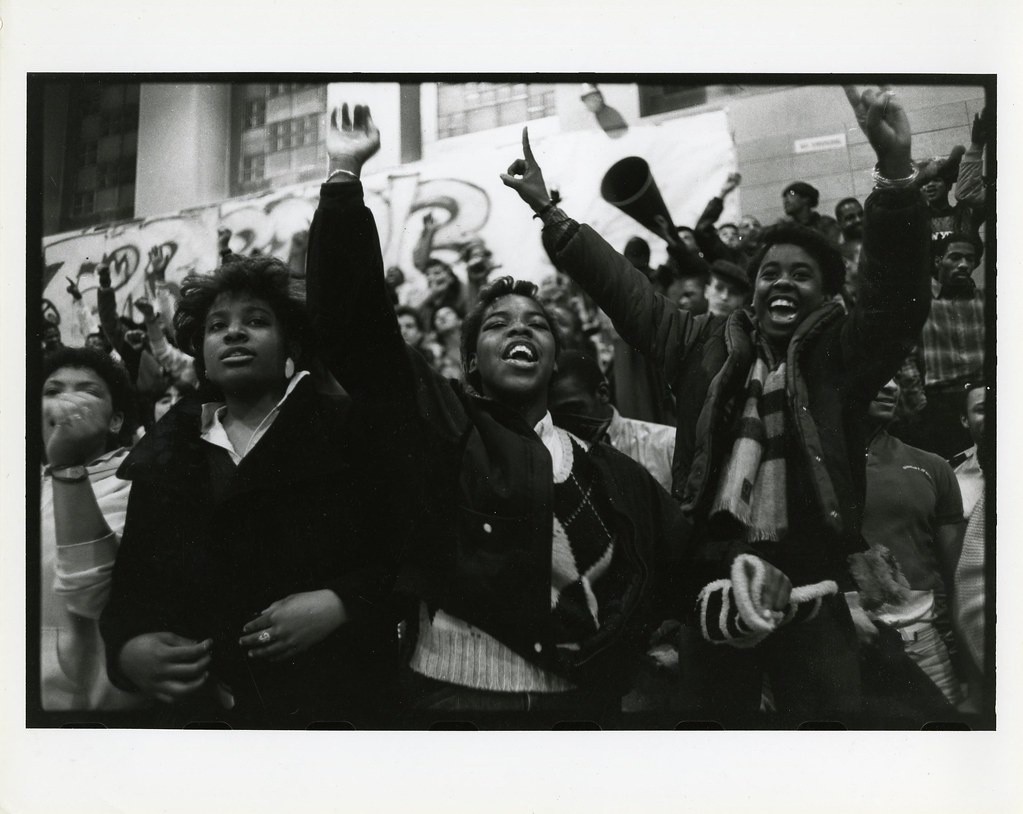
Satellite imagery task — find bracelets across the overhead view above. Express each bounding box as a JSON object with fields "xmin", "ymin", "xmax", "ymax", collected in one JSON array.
[
  {"xmin": 873, "ymin": 158, "xmax": 919, "ymax": 190},
  {"xmin": 326, "ymin": 169, "xmax": 359, "ymax": 183},
  {"xmin": 533, "ymin": 190, "xmax": 562, "ymax": 219}
]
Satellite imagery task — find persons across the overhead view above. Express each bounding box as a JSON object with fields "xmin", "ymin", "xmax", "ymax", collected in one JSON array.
[{"xmin": 31, "ymin": 83, "xmax": 988, "ymax": 714}]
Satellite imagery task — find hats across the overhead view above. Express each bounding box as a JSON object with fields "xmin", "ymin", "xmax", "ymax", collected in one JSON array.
[
  {"xmin": 783, "ymin": 183, "xmax": 820, "ymax": 208},
  {"xmin": 709, "ymin": 259, "xmax": 754, "ymax": 293}
]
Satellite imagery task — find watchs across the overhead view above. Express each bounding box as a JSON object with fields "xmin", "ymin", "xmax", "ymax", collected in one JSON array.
[{"xmin": 44, "ymin": 462, "xmax": 88, "ymax": 483}]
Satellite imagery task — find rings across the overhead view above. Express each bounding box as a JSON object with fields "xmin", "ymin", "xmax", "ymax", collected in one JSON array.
[{"xmin": 258, "ymin": 630, "xmax": 271, "ymax": 645}]
